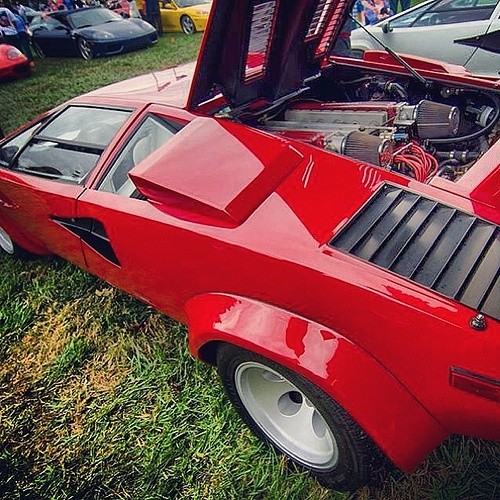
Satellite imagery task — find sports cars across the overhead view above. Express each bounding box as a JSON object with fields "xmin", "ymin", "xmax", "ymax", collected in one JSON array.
[
  {"xmin": 0, "ymin": 0, "xmax": 500, "ymax": 489},
  {"xmin": 157, "ymin": 0, "xmax": 213, "ymax": 35},
  {"xmin": 30, "ymin": 6, "xmax": 157, "ymax": 60},
  {"xmin": 0, "ymin": 40, "xmax": 32, "ymax": 84}
]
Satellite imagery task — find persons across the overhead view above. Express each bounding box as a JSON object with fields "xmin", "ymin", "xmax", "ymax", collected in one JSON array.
[
  {"xmin": 11, "ymin": 13, "xmax": 35, "ymax": 68},
  {"xmin": 342, "ymin": 1, "xmax": 366, "ymax": 34},
  {"xmin": 144, "ymin": 0, "xmax": 164, "ymax": 36},
  {"xmin": 389, "ymin": 0, "xmax": 411, "ymax": 19},
  {"xmin": 0, "ymin": 0, "xmax": 130, "ymax": 26},
  {"xmin": 0, "ymin": 26, "xmax": 23, "ymax": 53},
  {"xmin": 361, "ymin": 0, "xmax": 394, "ymax": 25}
]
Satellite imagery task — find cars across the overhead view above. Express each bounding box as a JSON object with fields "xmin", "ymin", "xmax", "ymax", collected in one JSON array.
[{"xmin": 351, "ymin": 1, "xmax": 500, "ymax": 77}]
